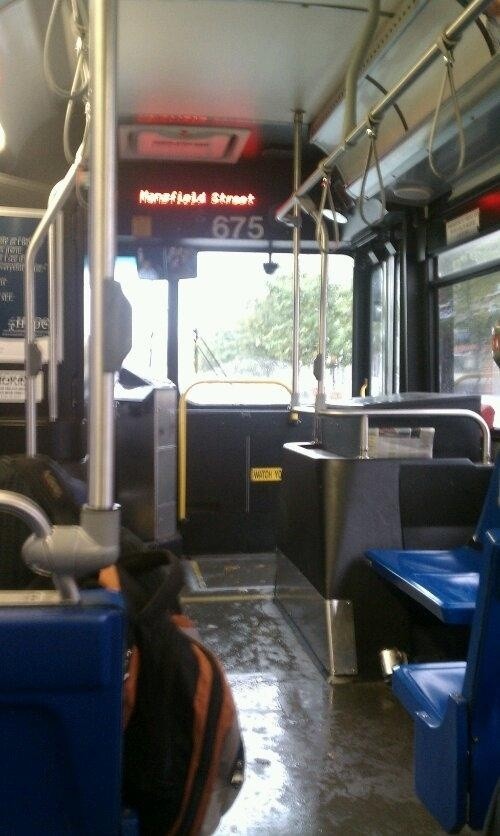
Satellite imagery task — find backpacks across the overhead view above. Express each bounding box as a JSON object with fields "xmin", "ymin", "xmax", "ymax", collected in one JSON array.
[{"xmin": 96, "ymin": 527, "xmax": 246, "ymax": 836}]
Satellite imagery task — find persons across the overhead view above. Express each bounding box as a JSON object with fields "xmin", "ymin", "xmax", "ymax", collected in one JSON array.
[{"xmin": 138, "ymin": 247, "xmax": 197, "ymax": 278}]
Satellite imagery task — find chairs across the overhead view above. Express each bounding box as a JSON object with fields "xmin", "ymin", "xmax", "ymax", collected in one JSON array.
[
  {"xmin": 2, "ymin": 593, "xmax": 125, "ymax": 835},
  {"xmin": 363, "ymin": 445, "xmax": 497, "ymax": 627},
  {"xmin": 390, "ymin": 530, "xmax": 500, "ymax": 836}
]
